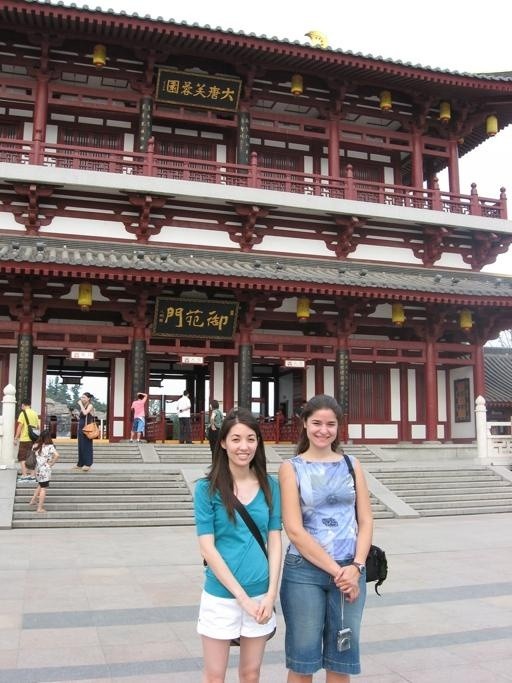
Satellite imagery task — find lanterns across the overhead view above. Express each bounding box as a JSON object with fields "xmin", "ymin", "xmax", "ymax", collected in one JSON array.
[
  {"xmin": 290, "ymin": 74, "xmax": 304, "ymax": 96},
  {"xmin": 379, "ymin": 90, "xmax": 392, "ymax": 112},
  {"xmin": 297, "ymin": 297, "xmax": 310, "ymax": 320},
  {"xmin": 78, "ymin": 281, "xmax": 92, "ymax": 312},
  {"xmin": 93, "ymin": 44, "xmax": 106, "ymax": 68},
  {"xmin": 460, "ymin": 311, "xmax": 472, "ymax": 330},
  {"xmin": 392, "ymin": 302, "xmax": 404, "ymax": 325},
  {"xmin": 440, "ymin": 102, "xmax": 451, "ymax": 123},
  {"xmin": 486, "ymin": 115, "xmax": 498, "ymax": 137}
]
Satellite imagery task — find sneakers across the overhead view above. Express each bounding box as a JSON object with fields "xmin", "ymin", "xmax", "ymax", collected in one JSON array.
[
  {"xmin": 37, "ymin": 508, "xmax": 47, "ymax": 512},
  {"xmin": 25, "ymin": 475, "xmax": 37, "ymax": 482},
  {"xmin": 71, "ymin": 464, "xmax": 82, "ymax": 469},
  {"xmin": 129, "ymin": 440, "xmax": 134, "ymax": 444},
  {"xmin": 16, "ymin": 475, "xmax": 27, "ymax": 482},
  {"xmin": 82, "ymin": 464, "xmax": 92, "ymax": 471},
  {"xmin": 136, "ymin": 440, "xmax": 143, "ymax": 444},
  {"xmin": 29, "ymin": 500, "xmax": 39, "ymax": 505}
]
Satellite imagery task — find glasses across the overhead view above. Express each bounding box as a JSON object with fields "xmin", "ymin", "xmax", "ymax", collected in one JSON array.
[{"xmin": 81, "ymin": 397, "xmax": 88, "ymax": 399}]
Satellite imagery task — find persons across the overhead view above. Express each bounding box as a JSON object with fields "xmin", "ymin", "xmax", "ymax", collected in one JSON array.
[
  {"xmin": 129, "ymin": 392, "xmax": 148, "ymax": 443},
  {"xmin": 176, "ymin": 389, "xmax": 192, "ymax": 443},
  {"xmin": 294, "ymin": 399, "xmax": 309, "ymax": 419},
  {"xmin": 279, "ymin": 395, "xmax": 374, "ymax": 683},
  {"xmin": 14, "ymin": 392, "xmax": 100, "ymax": 513},
  {"xmin": 193, "ymin": 410, "xmax": 283, "ymax": 683},
  {"xmin": 206, "ymin": 400, "xmax": 223, "ymax": 469}
]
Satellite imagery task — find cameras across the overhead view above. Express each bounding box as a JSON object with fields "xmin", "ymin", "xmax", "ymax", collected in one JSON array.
[{"xmin": 338, "ymin": 628, "xmax": 354, "ymax": 654}]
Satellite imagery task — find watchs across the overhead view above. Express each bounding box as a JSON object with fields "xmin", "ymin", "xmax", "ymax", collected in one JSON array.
[{"xmin": 351, "ymin": 562, "xmax": 367, "ymax": 573}]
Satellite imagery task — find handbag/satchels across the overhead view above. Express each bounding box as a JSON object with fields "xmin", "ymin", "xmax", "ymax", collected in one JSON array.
[
  {"xmin": 28, "ymin": 425, "xmax": 40, "ymax": 440},
  {"xmin": 25, "ymin": 449, "xmax": 37, "ymax": 470},
  {"xmin": 82, "ymin": 422, "xmax": 100, "ymax": 439},
  {"xmin": 366, "ymin": 545, "xmax": 388, "ymax": 597}
]
